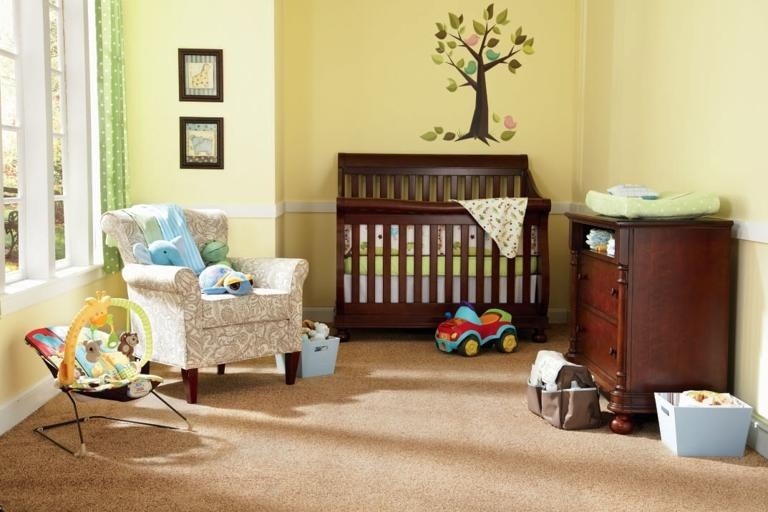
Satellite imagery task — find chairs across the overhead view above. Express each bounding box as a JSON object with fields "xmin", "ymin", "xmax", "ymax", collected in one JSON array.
[
  {"xmin": 25, "ymin": 297, "xmax": 194, "ymax": 458},
  {"xmin": 100, "ymin": 207, "xmax": 310, "ymax": 404}
]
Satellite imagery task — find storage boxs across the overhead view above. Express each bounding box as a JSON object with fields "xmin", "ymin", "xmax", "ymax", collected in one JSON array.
[
  {"xmin": 654, "ymin": 391, "xmax": 754, "ymax": 458},
  {"xmin": 274, "ymin": 336, "xmax": 340, "ymax": 378}
]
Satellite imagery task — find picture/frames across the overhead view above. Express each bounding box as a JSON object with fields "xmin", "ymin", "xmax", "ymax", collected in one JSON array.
[
  {"xmin": 178, "ymin": 48, "xmax": 223, "ymax": 102},
  {"xmin": 179, "ymin": 116, "xmax": 224, "ymax": 169}
]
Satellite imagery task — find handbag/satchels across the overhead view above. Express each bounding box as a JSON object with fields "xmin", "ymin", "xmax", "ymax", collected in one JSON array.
[{"xmin": 527, "ymin": 348, "xmax": 604, "ymax": 430}]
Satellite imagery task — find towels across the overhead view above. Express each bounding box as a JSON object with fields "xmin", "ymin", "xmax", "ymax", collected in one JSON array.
[{"xmin": 103, "ymin": 204, "xmax": 206, "ymax": 276}]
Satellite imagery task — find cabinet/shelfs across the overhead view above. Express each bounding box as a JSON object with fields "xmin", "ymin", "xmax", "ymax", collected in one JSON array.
[{"xmin": 562, "ymin": 211, "xmax": 735, "ymax": 436}]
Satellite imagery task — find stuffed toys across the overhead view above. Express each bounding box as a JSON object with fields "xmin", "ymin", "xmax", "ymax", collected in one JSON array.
[
  {"xmin": 57, "ymin": 289, "xmax": 153, "ymax": 387},
  {"xmin": 200, "ymin": 238, "xmax": 241, "ymax": 273},
  {"xmin": 199, "ymin": 263, "xmax": 255, "ymax": 295},
  {"xmin": 132, "ymin": 236, "xmax": 188, "ymax": 265},
  {"xmin": 302, "ymin": 320, "xmax": 331, "ymax": 351}
]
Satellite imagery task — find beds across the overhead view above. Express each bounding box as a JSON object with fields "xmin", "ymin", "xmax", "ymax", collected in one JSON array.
[{"xmin": 330, "ymin": 151, "xmax": 552, "ymax": 343}]
